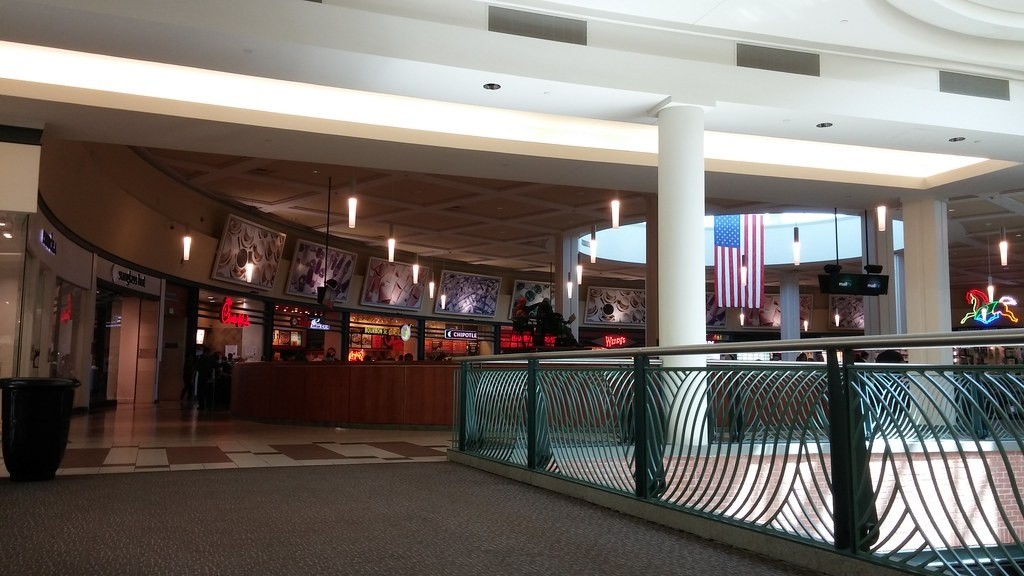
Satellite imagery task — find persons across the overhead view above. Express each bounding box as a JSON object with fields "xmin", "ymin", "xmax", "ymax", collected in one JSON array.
[
  {"xmin": 718, "ymin": 349, "xmax": 909, "ymax": 363},
  {"xmin": 533, "ymin": 298, "xmax": 583, "ymax": 348},
  {"xmin": 511, "ymin": 295, "xmax": 544, "ymax": 347},
  {"xmin": 179, "ymin": 348, "xmax": 412, "ymax": 411}
]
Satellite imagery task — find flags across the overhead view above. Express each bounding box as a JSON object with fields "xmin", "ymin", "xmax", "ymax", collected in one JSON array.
[{"xmin": 714, "ymin": 216, "xmax": 765, "ymax": 309}]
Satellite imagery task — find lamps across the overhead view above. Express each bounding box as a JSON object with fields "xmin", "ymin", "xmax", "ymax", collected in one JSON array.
[
  {"xmin": 182, "ymin": 235, "xmax": 193, "ymax": 261},
  {"xmin": 386, "ymin": 224, "xmax": 397, "ymax": 262},
  {"xmin": 999, "ymin": 226, "xmax": 1008, "ymax": 266},
  {"xmin": 611, "ymin": 192, "xmax": 621, "ymax": 230},
  {"xmin": 428, "ymin": 269, "xmax": 435, "ymax": 298},
  {"xmin": 412, "ymin": 252, "xmax": 420, "ymax": 284},
  {"xmin": 987, "ymin": 274, "xmax": 993, "ymax": 302},
  {"xmin": 567, "ymin": 272, "xmax": 573, "ymax": 299},
  {"xmin": 441, "ymin": 284, "xmax": 446, "ymax": 309},
  {"xmin": 793, "ymin": 227, "xmax": 800, "ymax": 266},
  {"xmin": 577, "ymin": 254, "xmax": 582, "ymax": 285},
  {"xmin": 877, "ymin": 205, "xmax": 886, "ymax": 232},
  {"xmin": 348, "ymin": 173, "xmax": 358, "ymax": 228},
  {"xmin": 590, "ymin": 223, "xmax": 597, "ymax": 263}
]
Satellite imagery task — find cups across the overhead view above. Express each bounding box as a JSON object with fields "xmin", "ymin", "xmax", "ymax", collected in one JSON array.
[
  {"xmin": 587, "ymin": 288, "xmax": 645, "ymax": 323},
  {"xmin": 213, "ymin": 219, "xmax": 285, "ymax": 286}
]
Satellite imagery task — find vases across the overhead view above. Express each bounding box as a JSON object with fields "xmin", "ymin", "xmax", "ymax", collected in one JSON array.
[{"xmin": 0, "ymin": 377, "xmax": 82, "ymax": 483}]
